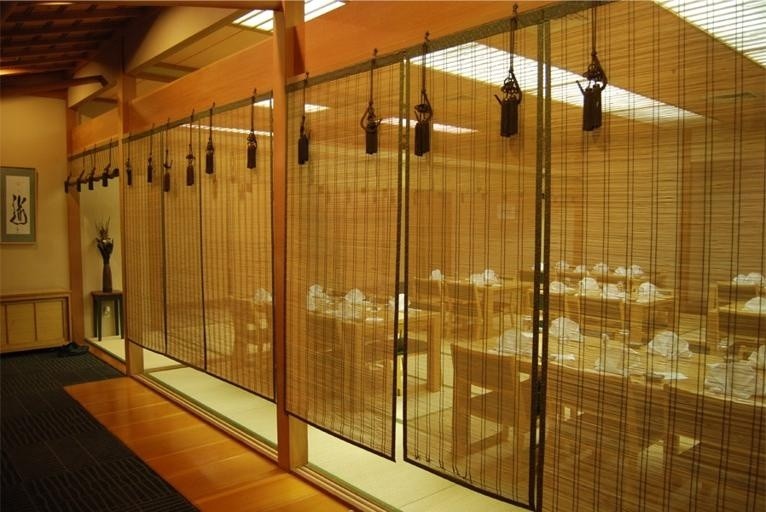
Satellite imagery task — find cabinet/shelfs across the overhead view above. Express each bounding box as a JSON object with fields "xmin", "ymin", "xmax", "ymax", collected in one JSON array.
[{"xmin": 0, "ymin": 285, "xmax": 72, "ymax": 353}]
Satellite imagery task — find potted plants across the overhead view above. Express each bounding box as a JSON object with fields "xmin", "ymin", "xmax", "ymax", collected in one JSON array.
[{"xmin": 93, "ymin": 216, "xmax": 114, "ymax": 291}]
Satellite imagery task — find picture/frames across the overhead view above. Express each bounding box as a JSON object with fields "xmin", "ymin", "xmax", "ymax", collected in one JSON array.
[{"xmin": 0, "ymin": 162, "xmax": 40, "ymax": 244}]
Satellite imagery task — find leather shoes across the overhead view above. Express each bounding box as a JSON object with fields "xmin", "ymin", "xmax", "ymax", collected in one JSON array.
[
  {"xmin": 56, "ymin": 348, "xmax": 86, "ymax": 357},
  {"xmin": 61, "ymin": 342, "xmax": 88, "ymax": 350}
]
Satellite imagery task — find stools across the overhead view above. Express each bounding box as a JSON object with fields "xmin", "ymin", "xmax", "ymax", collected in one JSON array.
[{"xmin": 90, "ymin": 291, "xmax": 124, "ymax": 341}]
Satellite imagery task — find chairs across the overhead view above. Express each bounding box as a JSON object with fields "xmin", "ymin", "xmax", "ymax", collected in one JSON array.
[{"xmin": 222, "ymin": 259, "xmax": 765, "ymax": 511}]
